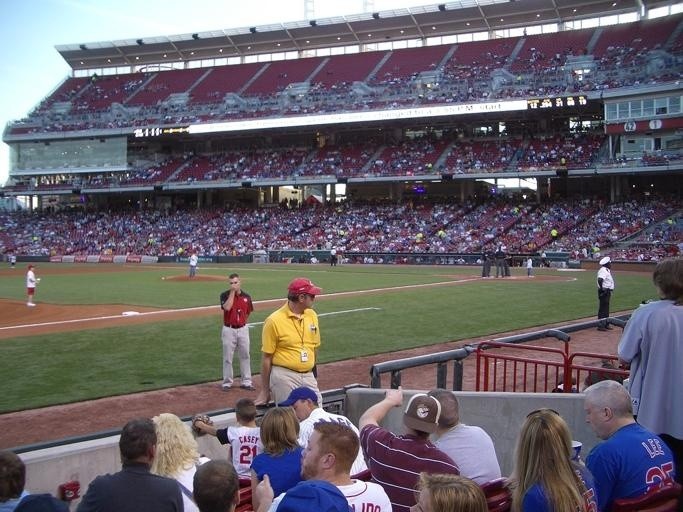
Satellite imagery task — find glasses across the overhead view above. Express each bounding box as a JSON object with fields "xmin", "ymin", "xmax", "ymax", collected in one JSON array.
[{"xmin": 526, "ymin": 408, "xmax": 559, "ymax": 416}]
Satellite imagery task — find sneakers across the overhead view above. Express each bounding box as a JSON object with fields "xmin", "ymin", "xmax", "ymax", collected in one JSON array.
[{"xmin": 222, "ymin": 379, "xmax": 256, "ymax": 390}]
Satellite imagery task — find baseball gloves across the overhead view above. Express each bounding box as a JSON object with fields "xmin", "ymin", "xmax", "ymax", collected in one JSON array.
[{"xmin": 191, "ymin": 413, "xmax": 213, "ymax": 430}]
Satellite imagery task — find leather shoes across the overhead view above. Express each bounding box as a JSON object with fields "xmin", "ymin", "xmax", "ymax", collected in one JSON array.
[{"xmin": 597, "ymin": 326, "xmax": 613, "ymax": 331}]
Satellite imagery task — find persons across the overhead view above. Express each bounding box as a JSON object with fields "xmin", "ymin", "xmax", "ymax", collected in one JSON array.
[
  {"xmin": 0, "ymin": 376, "xmax": 682, "ymax": 510},
  {"xmin": 218, "ymin": 272, "xmax": 256, "ymax": 392},
  {"xmin": 253, "ymin": 277, "xmax": 323, "ymax": 410},
  {"xmin": 618, "ymin": 256, "xmax": 681, "ymax": 480},
  {"xmin": 595, "ymin": 255, "xmax": 614, "ymax": 332},
  {"xmin": 24, "ymin": 263, "xmax": 41, "ymax": 309},
  {"xmin": 1, "ymin": 35, "xmax": 683, "ymax": 280}
]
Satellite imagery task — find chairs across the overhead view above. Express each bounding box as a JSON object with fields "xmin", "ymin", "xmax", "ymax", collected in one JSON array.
[
  {"xmin": 609, "ymin": 479, "xmax": 681, "ymax": 512},
  {"xmin": 350, "ymin": 468, "xmax": 372, "ymax": 483},
  {"xmin": 479, "ymin": 477, "xmax": 515, "ymax": 511},
  {"xmin": 233, "ymin": 478, "xmax": 254, "ymax": 510}
]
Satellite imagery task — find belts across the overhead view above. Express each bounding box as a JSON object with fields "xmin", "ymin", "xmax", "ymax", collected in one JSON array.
[{"xmin": 226, "ymin": 324, "xmax": 245, "ymax": 328}]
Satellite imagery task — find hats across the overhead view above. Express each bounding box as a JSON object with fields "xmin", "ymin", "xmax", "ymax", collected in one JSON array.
[
  {"xmin": 278, "ymin": 386, "xmax": 317, "ymax": 406},
  {"xmin": 599, "ymin": 256, "xmax": 611, "ymax": 265},
  {"xmin": 403, "ymin": 393, "xmax": 442, "ymax": 433},
  {"xmin": 288, "ymin": 278, "xmax": 322, "ymax": 295}
]
